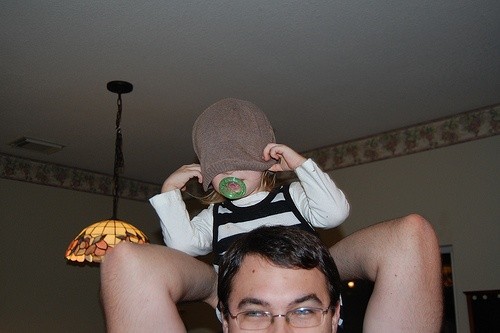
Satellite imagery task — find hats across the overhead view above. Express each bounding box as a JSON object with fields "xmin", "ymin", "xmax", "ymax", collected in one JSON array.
[{"xmin": 191, "ymin": 98, "xmax": 279, "ymax": 192}]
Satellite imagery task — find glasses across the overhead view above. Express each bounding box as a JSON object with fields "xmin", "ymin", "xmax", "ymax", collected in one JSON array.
[{"xmin": 220, "ymin": 300, "xmax": 336, "ymax": 331}]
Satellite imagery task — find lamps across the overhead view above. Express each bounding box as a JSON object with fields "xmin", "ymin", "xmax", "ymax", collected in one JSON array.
[{"xmin": 66, "ymin": 83, "xmax": 150, "ymax": 265}]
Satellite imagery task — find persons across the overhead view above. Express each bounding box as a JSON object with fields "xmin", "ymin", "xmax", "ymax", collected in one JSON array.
[
  {"xmin": 100, "ymin": 214, "xmax": 443, "ymax": 333},
  {"xmin": 149, "ymin": 98, "xmax": 350, "ymax": 333}
]
